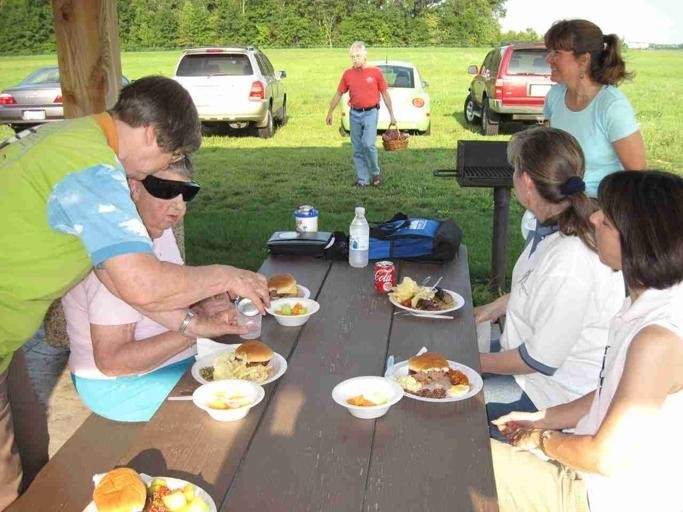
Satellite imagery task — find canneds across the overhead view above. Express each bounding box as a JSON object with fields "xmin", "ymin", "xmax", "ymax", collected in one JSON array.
[{"xmin": 373, "ymin": 261, "xmax": 396, "ymax": 294}]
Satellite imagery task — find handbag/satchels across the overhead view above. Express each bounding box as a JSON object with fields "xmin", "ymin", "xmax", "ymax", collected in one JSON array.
[{"xmin": 368, "ymin": 212, "xmax": 463, "ymax": 258}]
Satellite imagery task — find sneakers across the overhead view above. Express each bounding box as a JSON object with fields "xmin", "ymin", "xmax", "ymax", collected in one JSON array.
[
  {"xmin": 373, "ymin": 175, "xmax": 381, "ymax": 186},
  {"xmin": 351, "ymin": 182, "xmax": 369, "ymax": 187}
]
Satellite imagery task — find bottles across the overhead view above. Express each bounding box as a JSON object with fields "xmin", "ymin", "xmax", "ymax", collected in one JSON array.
[{"xmin": 349, "ymin": 206, "xmax": 370, "ymax": 268}]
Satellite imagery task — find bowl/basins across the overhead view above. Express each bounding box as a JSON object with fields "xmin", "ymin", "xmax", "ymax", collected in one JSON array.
[
  {"xmin": 266, "ymin": 298, "xmax": 320, "ymax": 327},
  {"xmin": 330, "ymin": 373, "xmax": 404, "ymax": 422},
  {"xmin": 191, "ymin": 378, "xmax": 266, "ymax": 422},
  {"xmin": 296, "ymin": 283, "xmax": 310, "ymax": 298}
]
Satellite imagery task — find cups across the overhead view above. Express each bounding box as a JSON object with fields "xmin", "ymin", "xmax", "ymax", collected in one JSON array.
[{"xmin": 234, "ymin": 302, "xmax": 262, "ymax": 340}]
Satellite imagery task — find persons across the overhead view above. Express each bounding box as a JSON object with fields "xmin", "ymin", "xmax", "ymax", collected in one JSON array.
[
  {"xmin": 0, "ymin": 75, "xmax": 273, "ymax": 511},
  {"xmin": 487, "ymin": 169, "xmax": 683, "ymax": 510},
  {"xmin": 60, "ymin": 153, "xmax": 238, "ymax": 424},
  {"xmin": 538, "ymin": 17, "xmax": 648, "ymax": 202},
  {"xmin": 470, "ymin": 125, "xmax": 628, "ymax": 443},
  {"xmin": 325, "ymin": 40, "xmax": 398, "ymax": 190}
]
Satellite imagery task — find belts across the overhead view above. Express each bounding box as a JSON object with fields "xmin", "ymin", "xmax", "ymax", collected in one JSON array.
[{"xmin": 351, "ymin": 106, "xmax": 377, "ymax": 111}]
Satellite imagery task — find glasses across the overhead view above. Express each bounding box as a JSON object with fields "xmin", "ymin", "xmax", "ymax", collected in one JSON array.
[{"xmin": 142, "ymin": 175, "xmax": 200, "ymax": 201}]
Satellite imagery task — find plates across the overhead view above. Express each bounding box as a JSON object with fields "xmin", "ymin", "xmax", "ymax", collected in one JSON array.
[
  {"xmin": 388, "ymin": 288, "xmax": 465, "ymax": 313},
  {"xmin": 384, "ymin": 357, "xmax": 484, "ymax": 404},
  {"xmin": 81, "ymin": 474, "xmax": 217, "ymax": 512},
  {"xmin": 191, "ymin": 345, "xmax": 288, "ymax": 385}
]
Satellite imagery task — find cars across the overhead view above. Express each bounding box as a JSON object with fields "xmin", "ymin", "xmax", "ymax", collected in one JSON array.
[
  {"xmin": 340, "ymin": 60, "xmax": 431, "ymax": 138},
  {"xmin": 0, "ymin": 65, "xmax": 133, "ymax": 133}
]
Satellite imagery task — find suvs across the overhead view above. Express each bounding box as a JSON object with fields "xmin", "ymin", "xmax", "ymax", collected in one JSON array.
[
  {"xmin": 464, "ymin": 44, "xmax": 580, "ymax": 135},
  {"xmin": 173, "ymin": 45, "xmax": 288, "ymax": 138}
]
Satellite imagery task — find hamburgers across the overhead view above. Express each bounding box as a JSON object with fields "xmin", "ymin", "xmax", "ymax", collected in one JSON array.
[
  {"xmin": 92, "ymin": 467, "xmax": 148, "ymax": 511},
  {"xmin": 408, "ymin": 352, "xmax": 450, "ymax": 381},
  {"xmin": 267, "ymin": 273, "xmax": 297, "ymax": 298},
  {"xmin": 234, "ymin": 339, "xmax": 273, "ymax": 369}
]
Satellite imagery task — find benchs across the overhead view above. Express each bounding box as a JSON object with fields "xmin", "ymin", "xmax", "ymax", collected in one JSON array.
[{"xmin": 0, "ymin": 411, "xmax": 145, "ymax": 512}]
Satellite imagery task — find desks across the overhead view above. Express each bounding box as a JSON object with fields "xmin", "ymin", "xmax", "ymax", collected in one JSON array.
[{"xmin": 111, "ymin": 234, "xmax": 499, "ymax": 511}]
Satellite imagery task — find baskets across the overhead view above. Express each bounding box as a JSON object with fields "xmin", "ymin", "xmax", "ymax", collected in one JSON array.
[{"xmin": 381, "ymin": 122, "xmax": 409, "ymax": 151}]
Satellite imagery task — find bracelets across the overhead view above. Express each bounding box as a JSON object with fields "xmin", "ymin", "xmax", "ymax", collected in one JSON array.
[
  {"xmin": 177, "ymin": 311, "xmax": 194, "ymax": 335},
  {"xmin": 538, "ymin": 428, "xmax": 554, "ymax": 457}
]
